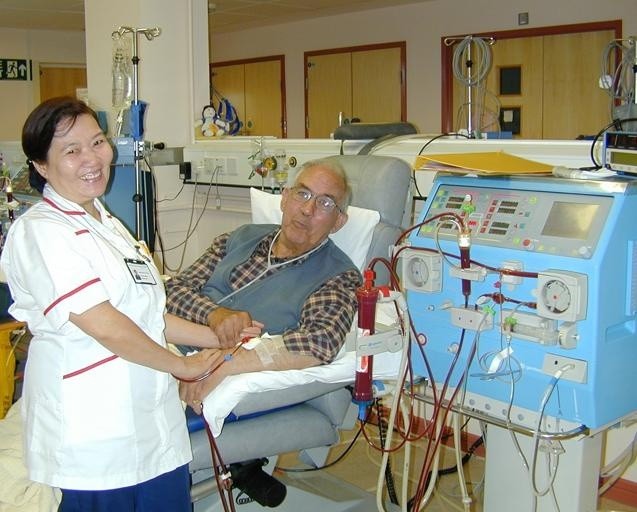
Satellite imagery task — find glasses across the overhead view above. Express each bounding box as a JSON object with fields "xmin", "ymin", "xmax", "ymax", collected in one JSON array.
[{"xmin": 290, "ymin": 187, "xmax": 343, "ymax": 216}]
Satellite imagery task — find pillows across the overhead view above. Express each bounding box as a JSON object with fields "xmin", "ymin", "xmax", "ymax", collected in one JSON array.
[{"xmin": 249, "ymin": 187, "xmax": 380, "ymax": 282}]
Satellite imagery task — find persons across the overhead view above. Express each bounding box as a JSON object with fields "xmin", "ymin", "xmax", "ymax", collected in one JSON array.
[
  {"xmin": 162, "ymin": 160, "xmax": 364, "ymax": 417},
  {"xmin": 0, "ymin": 96, "xmax": 265, "ymax": 509}
]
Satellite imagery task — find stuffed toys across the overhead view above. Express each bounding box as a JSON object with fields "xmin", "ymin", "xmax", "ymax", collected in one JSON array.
[{"xmin": 193, "ymin": 105, "xmax": 225, "ymax": 137}]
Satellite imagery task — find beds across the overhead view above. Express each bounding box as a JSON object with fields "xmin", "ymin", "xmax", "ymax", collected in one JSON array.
[{"xmin": 1, "ymin": 156, "xmax": 411, "ymax": 512}]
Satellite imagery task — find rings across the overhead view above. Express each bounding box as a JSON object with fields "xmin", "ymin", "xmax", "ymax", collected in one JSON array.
[{"xmin": 192, "ymin": 399, "xmax": 202, "ymax": 405}]
